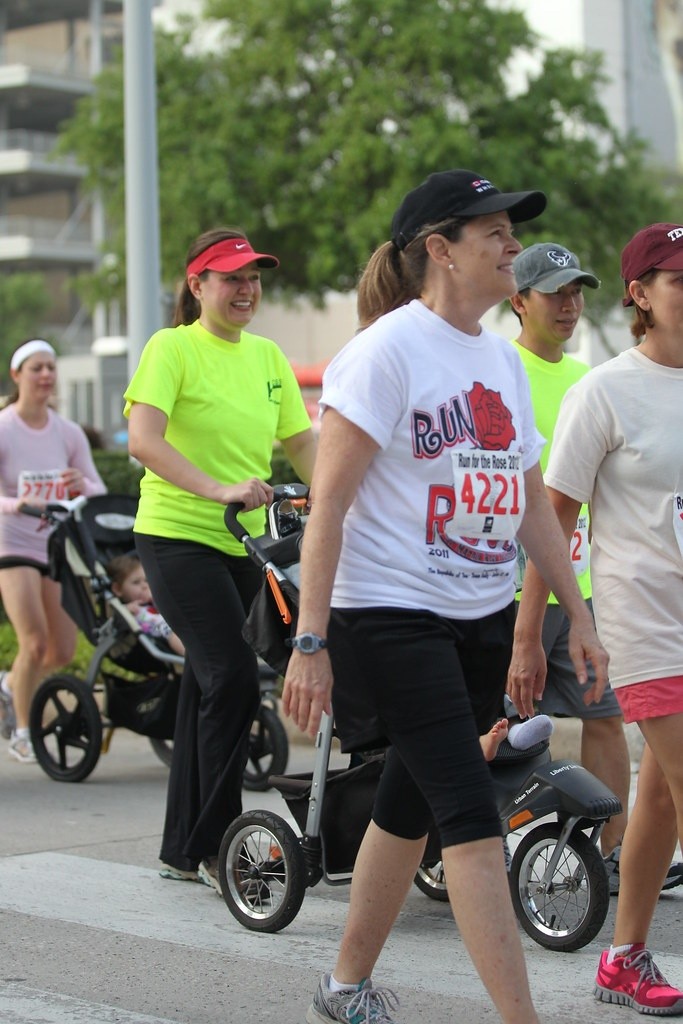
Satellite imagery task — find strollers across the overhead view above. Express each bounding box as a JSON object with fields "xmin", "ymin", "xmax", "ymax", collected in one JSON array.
[
  {"xmin": 219, "ymin": 482, "xmax": 623, "ymax": 951},
  {"xmin": 19, "ymin": 494, "xmax": 289, "ymax": 792}
]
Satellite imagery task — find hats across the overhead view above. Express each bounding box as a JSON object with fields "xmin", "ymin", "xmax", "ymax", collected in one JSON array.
[
  {"xmin": 511, "ymin": 240, "xmax": 599, "ymax": 294},
  {"xmin": 186, "ymin": 238, "xmax": 279, "ymax": 272},
  {"xmin": 391, "ymin": 171, "xmax": 548, "ymax": 250},
  {"xmin": 619, "ymin": 222, "xmax": 683, "ymax": 306}
]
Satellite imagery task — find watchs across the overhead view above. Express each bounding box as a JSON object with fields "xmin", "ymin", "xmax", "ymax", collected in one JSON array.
[{"xmin": 286, "ymin": 632, "xmax": 328, "ymax": 655}]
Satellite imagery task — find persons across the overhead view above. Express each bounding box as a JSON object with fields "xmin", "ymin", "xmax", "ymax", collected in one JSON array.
[
  {"xmin": 503, "ymin": 240, "xmax": 682, "ymax": 899},
  {"xmin": 506, "ymin": 223, "xmax": 683, "ymax": 1016},
  {"xmin": 122, "ymin": 224, "xmax": 320, "ymax": 903},
  {"xmin": 0, "ymin": 337, "xmax": 109, "ymax": 764},
  {"xmin": 478, "ymin": 689, "xmax": 554, "ymax": 760},
  {"xmin": 283, "ymin": 171, "xmax": 611, "ymax": 1023},
  {"xmin": 111, "ymin": 550, "xmax": 187, "ymax": 672}
]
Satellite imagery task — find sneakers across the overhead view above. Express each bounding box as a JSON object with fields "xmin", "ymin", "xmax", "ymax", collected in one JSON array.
[
  {"xmin": 307, "ymin": 970, "xmax": 397, "ymax": 1024},
  {"xmin": 198, "ymin": 852, "xmax": 270, "ymax": 901},
  {"xmin": 502, "ymin": 830, "xmax": 522, "ymax": 872},
  {"xmin": 599, "ymin": 841, "xmax": 683, "ymax": 893},
  {"xmin": 0, "ymin": 670, "xmax": 16, "ymax": 739},
  {"xmin": 160, "ymin": 860, "xmax": 201, "ymax": 885},
  {"xmin": 592, "ymin": 943, "xmax": 682, "ymax": 1016},
  {"xmin": 8, "ymin": 724, "xmax": 46, "ymax": 763}
]
